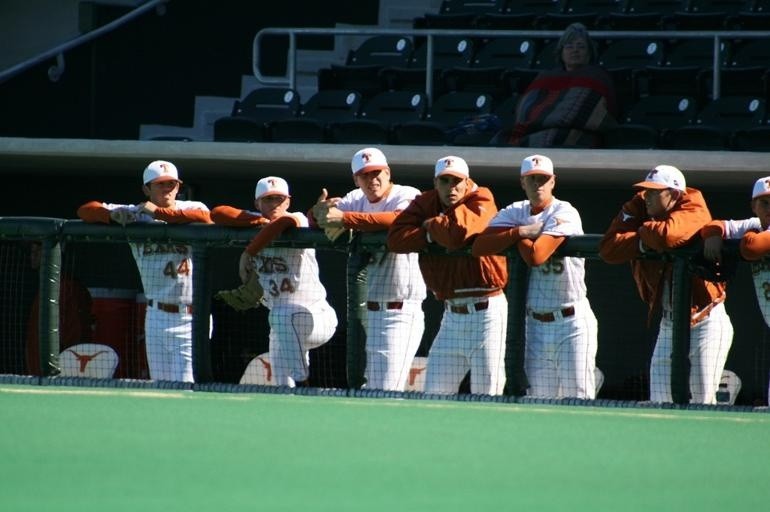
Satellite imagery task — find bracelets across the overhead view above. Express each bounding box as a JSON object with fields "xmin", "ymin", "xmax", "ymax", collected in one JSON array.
[
  {"xmin": 639, "ymin": 238, "xmax": 646, "ymax": 254},
  {"xmin": 426, "ymin": 231, "xmax": 433, "ymax": 244}
]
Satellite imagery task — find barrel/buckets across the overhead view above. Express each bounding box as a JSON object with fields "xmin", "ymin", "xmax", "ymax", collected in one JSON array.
[
  {"xmin": 135, "ymin": 293, "xmax": 148, "ymax": 380},
  {"xmin": 85, "ymin": 285, "xmax": 137, "ymax": 378}
]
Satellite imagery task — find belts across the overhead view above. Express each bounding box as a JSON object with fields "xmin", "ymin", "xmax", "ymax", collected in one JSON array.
[
  {"xmin": 444, "ymin": 300, "xmax": 487, "ymax": 312},
  {"xmin": 148, "ymin": 300, "xmax": 192, "ymax": 313},
  {"xmin": 527, "ymin": 307, "xmax": 573, "ymax": 321},
  {"xmin": 366, "ymin": 300, "xmax": 402, "ymax": 310}
]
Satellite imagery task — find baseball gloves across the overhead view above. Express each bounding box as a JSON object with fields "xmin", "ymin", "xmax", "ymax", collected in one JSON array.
[{"xmin": 218, "ymin": 271, "xmax": 264, "ymax": 309}]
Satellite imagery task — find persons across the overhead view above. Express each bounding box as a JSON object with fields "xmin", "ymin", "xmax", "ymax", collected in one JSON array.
[
  {"xmin": 25, "ymin": 239, "xmax": 95, "ymax": 378},
  {"xmin": 472, "ymin": 154, "xmax": 599, "ymax": 403},
  {"xmin": 506, "ymin": 23, "xmax": 609, "ymax": 149},
  {"xmin": 210, "ymin": 174, "xmax": 339, "ymax": 387},
  {"xmin": 77, "ymin": 159, "xmax": 216, "ymax": 385},
  {"xmin": 597, "ymin": 164, "xmax": 734, "ymax": 406},
  {"xmin": 308, "ymin": 148, "xmax": 428, "ymax": 393},
  {"xmin": 700, "ymin": 174, "xmax": 770, "ymax": 328},
  {"xmin": 385, "ymin": 155, "xmax": 511, "ymax": 394}
]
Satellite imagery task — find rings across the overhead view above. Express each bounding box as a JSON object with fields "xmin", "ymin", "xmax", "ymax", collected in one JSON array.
[{"xmin": 707, "ymin": 253, "xmax": 710, "ymax": 257}]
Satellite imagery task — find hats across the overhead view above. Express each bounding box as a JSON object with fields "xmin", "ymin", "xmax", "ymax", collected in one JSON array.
[
  {"xmin": 256, "ymin": 176, "xmax": 291, "ymax": 201},
  {"xmin": 520, "ymin": 155, "xmax": 553, "ymax": 177},
  {"xmin": 434, "ymin": 155, "xmax": 470, "ymax": 180},
  {"xmin": 351, "ymin": 147, "xmax": 388, "ymax": 175},
  {"xmin": 143, "ymin": 161, "xmax": 183, "ymax": 186},
  {"xmin": 752, "ymin": 177, "xmax": 770, "ymax": 198},
  {"xmin": 632, "ymin": 165, "xmax": 686, "ymax": 192}
]
